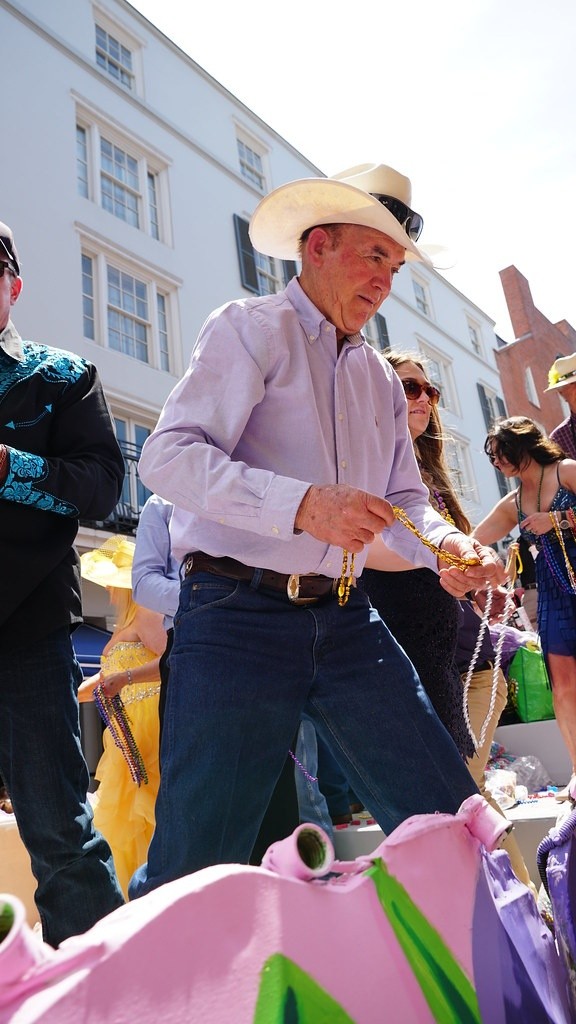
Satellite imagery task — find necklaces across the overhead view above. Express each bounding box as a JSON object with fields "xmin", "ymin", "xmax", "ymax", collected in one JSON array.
[
  {"xmin": 540, "ymin": 506, "xmax": 576, "ymax": 598},
  {"xmin": 462, "ymin": 577, "xmax": 510, "ymax": 750},
  {"xmin": 518, "ymin": 462, "xmax": 544, "ymax": 536},
  {"xmin": 333, "ymin": 498, "xmax": 482, "ymax": 605},
  {"xmin": 91, "ymin": 682, "xmax": 149, "ymax": 787}
]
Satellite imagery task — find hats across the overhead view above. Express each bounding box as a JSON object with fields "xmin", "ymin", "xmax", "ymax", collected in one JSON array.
[
  {"xmin": 1, "ymin": 221, "xmax": 19, "ymax": 274},
  {"xmin": 544, "ymin": 351, "xmax": 576, "ymax": 392},
  {"xmin": 249, "ymin": 163, "xmax": 457, "ymax": 270},
  {"xmin": 79, "ymin": 534, "xmax": 135, "ymax": 588}
]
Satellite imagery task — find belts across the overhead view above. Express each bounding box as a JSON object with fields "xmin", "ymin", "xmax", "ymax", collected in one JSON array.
[
  {"xmin": 525, "ymin": 583, "xmax": 538, "ymax": 589},
  {"xmin": 191, "ymin": 551, "xmax": 334, "ymax": 600}
]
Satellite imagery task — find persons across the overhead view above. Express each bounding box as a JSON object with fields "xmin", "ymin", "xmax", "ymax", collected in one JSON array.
[
  {"xmin": 131, "ymin": 158, "xmax": 483, "ymax": 899},
  {"xmin": 129, "ymin": 491, "xmax": 183, "ymax": 772},
  {"xmin": 456, "ymin": 596, "xmax": 510, "ymax": 788},
  {"xmin": 378, "ymin": 352, "xmax": 507, "ymax": 762},
  {"xmin": 75, "ymin": 536, "xmax": 171, "ymax": 902},
  {"xmin": 247, "ymin": 713, "xmax": 364, "ymax": 876},
  {"xmin": 546, "ymin": 350, "xmax": 576, "ymax": 458},
  {"xmin": 1, "ymin": 222, "xmax": 131, "ymax": 948},
  {"xmin": 470, "ymin": 415, "xmax": 576, "ymax": 802}
]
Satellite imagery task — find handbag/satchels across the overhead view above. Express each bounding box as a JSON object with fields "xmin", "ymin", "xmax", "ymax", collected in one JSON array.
[{"xmin": 511, "ymin": 646, "xmax": 554, "ymax": 723}]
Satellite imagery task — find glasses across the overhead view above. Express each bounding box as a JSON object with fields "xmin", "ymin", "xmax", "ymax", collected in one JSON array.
[
  {"xmin": 369, "ymin": 193, "xmax": 425, "ymax": 244},
  {"xmin": 0, "ymin": 260, "xmax": 16, "ymax": 279},
  {"xmin": 401, "ymin": 380, "xmax": 441, "ymax": 406},
  {"xmin": 480, "ymin": 593, "xmax": 507, "ymax": 600}
]
